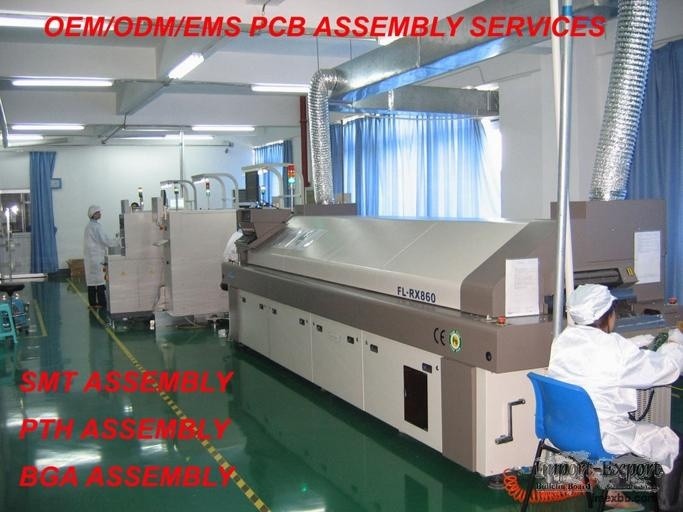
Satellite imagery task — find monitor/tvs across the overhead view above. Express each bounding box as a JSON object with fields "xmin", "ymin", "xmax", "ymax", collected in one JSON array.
[{"xmin": 245, "ymin": 171, "xmax": 260, "ymax": 202}]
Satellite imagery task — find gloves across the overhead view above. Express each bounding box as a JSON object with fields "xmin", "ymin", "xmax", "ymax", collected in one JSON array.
[
  {"xmin": 631, "ymin": 334, "xmax": 655, "ymax": 348},
  {"xmin": 668, "ymin": 328, "xmax": 683, "ymax": 344}
]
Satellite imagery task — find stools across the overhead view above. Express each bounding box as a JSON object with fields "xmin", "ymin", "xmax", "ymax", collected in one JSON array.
[{"xmin": 0, "ymin": 302, "xmax": 19, "ymax": 346}]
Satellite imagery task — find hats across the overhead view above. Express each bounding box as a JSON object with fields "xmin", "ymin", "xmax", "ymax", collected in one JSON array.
[
  {"xmin": 567, "ymin": 282, "xmax": 618, "ymax": 325},
  {"xmin": 88, "ymin": 206, "xmax": 101, "ymax": 218}
]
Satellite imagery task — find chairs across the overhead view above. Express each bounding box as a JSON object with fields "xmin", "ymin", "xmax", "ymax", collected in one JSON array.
[{"xmin": 521, "ymin": 371, "xmax": 655, "ymax": 512}]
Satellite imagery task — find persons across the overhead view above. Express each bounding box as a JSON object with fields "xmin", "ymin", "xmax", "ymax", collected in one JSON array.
[
  {"xmin": 83, "ymin": 204, "xmax": 119, "ymax": 308},
  {"xmin": 546, "ymin": 283, "xmax": 683, "ymax": 510}
]
